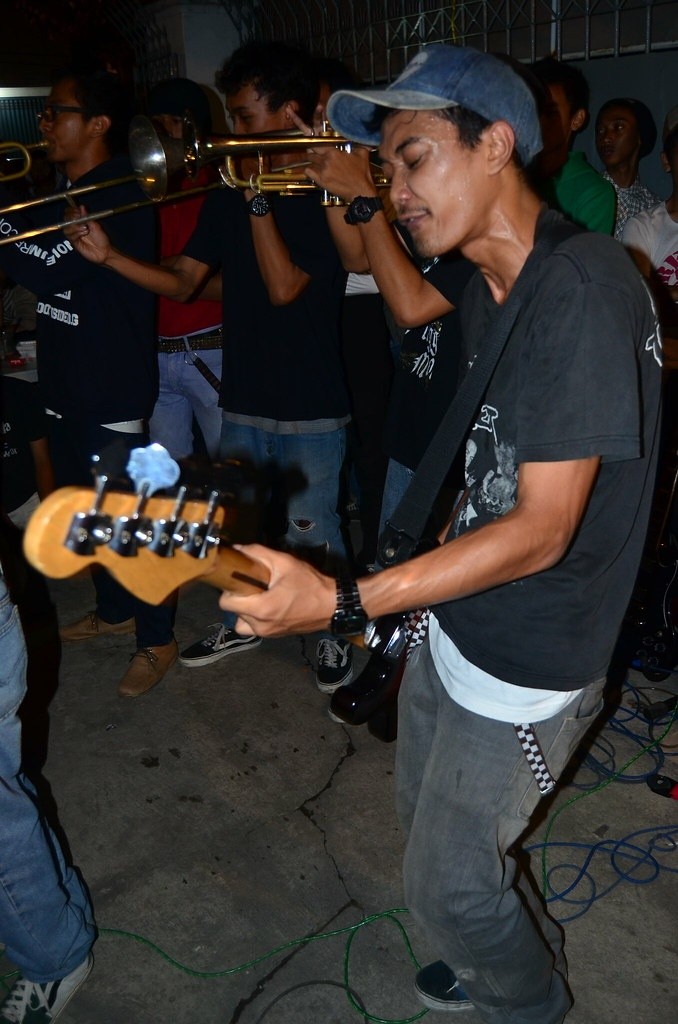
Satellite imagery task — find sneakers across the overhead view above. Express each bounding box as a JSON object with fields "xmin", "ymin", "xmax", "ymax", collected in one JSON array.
[
  {"xmin": 0, "ymin": 948, "xmax": 94, "ymax": 1024},
  {"xmin": 178, "ymin": 620, "xmax": 263, "ymax": 667},
  {"xmin": 118, "ymin": 638, "xmax": 178, "ymax": 698},
  {"xmin": 315, "ymin": 635, "xmax": 352, "ymax": 694},
  {"xmin": 57, "ymin": 610, "xmax": 137, "ymax": 642}
]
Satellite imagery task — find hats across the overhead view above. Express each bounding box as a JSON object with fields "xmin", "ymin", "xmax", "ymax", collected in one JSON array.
[{"xmin": 326, "ymin": 42, "xmax": 544, "ymax": 171}]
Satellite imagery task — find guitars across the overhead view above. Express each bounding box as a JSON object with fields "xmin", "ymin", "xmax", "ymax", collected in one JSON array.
[{"xmin": 24, "ymin": 438, "xmax": 421, "ymax": 744}]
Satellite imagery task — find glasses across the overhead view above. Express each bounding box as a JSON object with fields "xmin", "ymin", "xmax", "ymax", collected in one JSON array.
[{"xmin": 34, "ymin": 102, "xmax": 94, "ymax": 131}]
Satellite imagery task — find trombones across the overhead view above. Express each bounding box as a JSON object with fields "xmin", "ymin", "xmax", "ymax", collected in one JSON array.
[{"xmin": 1, "ymin": 124, "xmax": 304, "ymax": 243}]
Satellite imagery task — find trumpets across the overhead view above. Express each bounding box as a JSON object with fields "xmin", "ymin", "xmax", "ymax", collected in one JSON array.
[
  {"xmin": 128, "ymin": 116, "xmax": 391, "ymax": 208},
  {"xmin": 0, "ymin": 138, "xmax": 50, "ymax": 182}
]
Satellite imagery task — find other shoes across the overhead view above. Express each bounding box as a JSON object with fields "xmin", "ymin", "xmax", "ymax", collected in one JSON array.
[{"xmin": 414, "ymin": 960, "xmax": 476, "ymax": 1011}]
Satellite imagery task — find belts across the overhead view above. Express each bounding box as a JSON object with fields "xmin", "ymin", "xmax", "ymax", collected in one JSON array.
[{"xmin": 157, "ymin": 329, "xmax": 225, "ymax": 354}]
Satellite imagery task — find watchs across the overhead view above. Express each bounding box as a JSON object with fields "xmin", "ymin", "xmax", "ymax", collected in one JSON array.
[
  {"xmin": 331, "ymin": 577, "xmax": 368, "ymax": 639},
  {"xmin": 246, "ymin": 194, "xmax": 271, "ymax": 217},
  {"xmin": 344, "ymin": 196, "xmax": 384, "ymax": 225}
]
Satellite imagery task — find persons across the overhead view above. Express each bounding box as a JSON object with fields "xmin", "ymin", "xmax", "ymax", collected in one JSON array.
[
  {"xmin": 0, "ymin": 375, "xmax": 96, "ymax": 1024},
  {"xmin": 527, "ymin": 60, "xmax": 678, "ymax": 682},
  {"xmin": 285, "ymin": 108, "xmax": 476, "ymax": 724},
  {"xmin": 145, "ymin": 77, "xmax": 225, "ymax": 469},
  {"xmin": 219, "ymin": 49, "xmax": 661, "ymax": 1024},
  {"xmin": 65, "ymin": 38, "xmax": 358, "ymax": 694},
  {"xmin": 0, "ymin": 76, "xmax": 180, "ymax": 699}
]
